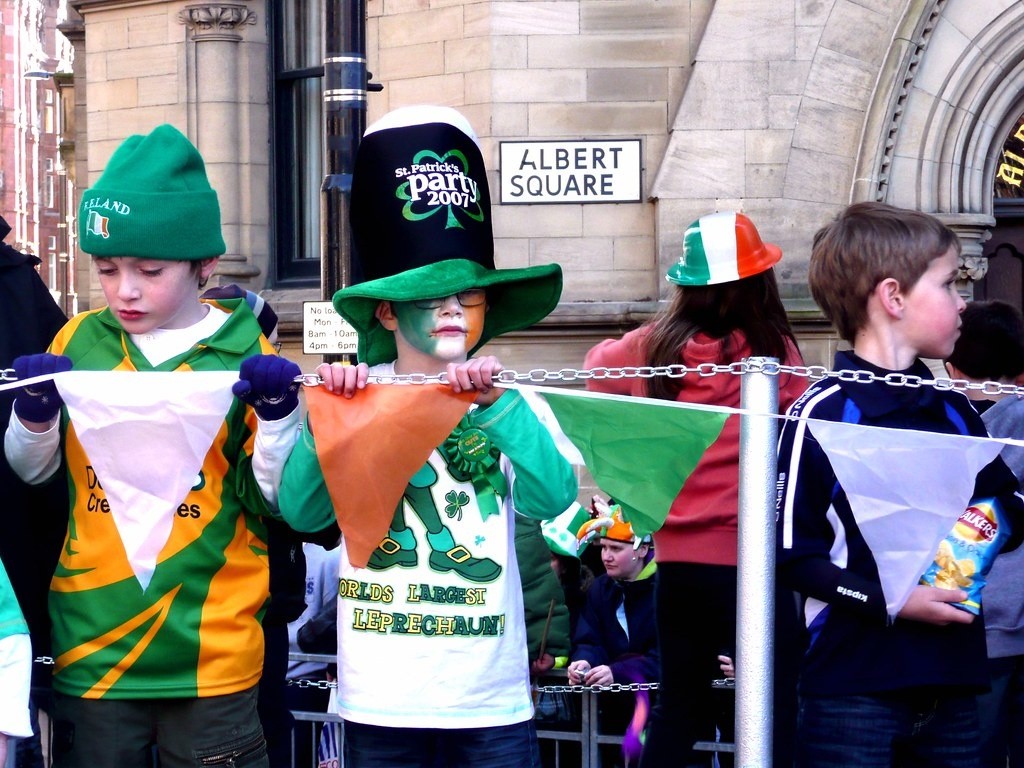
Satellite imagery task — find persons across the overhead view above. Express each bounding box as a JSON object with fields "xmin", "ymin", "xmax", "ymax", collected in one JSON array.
[
  {"xmin": 774, "ymin": 203, "xmax": 1024, "ymax": 768},
  {"xmin": 942, "ymin": 298, "xmax": 1024, "ymax": 768},
  {"xmin": 584, "ymin": 212, "xmax": 810, "ymax": 768},
  {"xmin": 3, "ymin": 124, "xmax": 308, "ymax": 768},
  {"xmin": 286, "ymin": 495, "xmax": 734, "ymax": 768},
  {"xmin": 0, "ymin": 209, "xmax": 77, "ymax": 768},
  {"xmin": 273, "ymin": 121, "xmax": 580, "ymax": 768}
]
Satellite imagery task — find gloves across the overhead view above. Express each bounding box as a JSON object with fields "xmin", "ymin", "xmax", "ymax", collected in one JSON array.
[
  {"xmin": 14, "ymin": 352, "xmax": 70, "ymax": 421},
  {"xmin": 233, "ymin": 354, "xmax": 301, "ymax": 422}
]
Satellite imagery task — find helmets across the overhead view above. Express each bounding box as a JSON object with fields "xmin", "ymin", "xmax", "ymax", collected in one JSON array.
[{"xmin": 663, "ymin": 211, "xmax": 783, "ymax": 286}]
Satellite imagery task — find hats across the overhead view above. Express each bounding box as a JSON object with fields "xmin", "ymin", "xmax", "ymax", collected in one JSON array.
[
  {"xmin": 76, "ymin": 124, "xmax": 226, "ymax": 261},
  {"xmin": 540, "ymin": 501, "xmax": 597, "ymax": 578},
  {"xmin": 198, "ymin": 284, "xmax": 278, "ymax": 340},
  {"xmin": 332, "ymin": 106, "xmax": 563, "ymax": 366},
  {"xmin": 576, "ymin": 504, "xmax": 651, "ymax": 551}
]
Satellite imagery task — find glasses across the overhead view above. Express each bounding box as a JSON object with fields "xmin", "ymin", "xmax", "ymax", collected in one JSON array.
[
  {"xmin": 414, "ymin": 288, "xmax": 487, "ymax": 309},
  {"xmin": 272, "ymin": 342, "xmax": 282, "ymax": 353}
]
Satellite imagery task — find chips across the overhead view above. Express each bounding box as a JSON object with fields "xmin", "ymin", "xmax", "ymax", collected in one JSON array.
[{"xmin": 934, "ymin": 540, "xmax": 976, "ymax": 590}]
